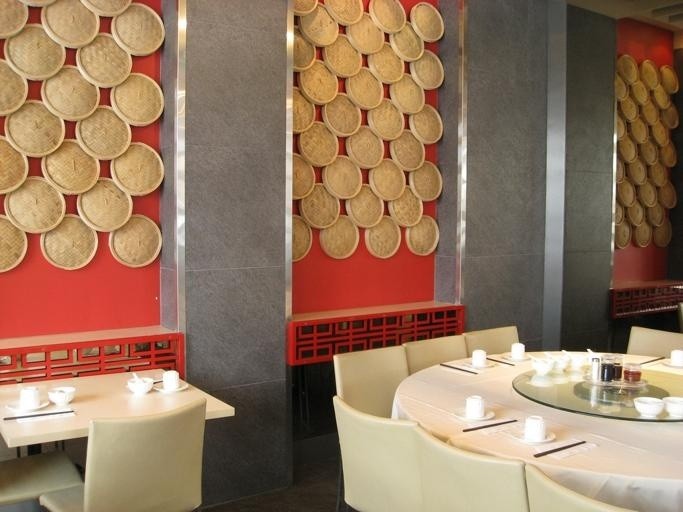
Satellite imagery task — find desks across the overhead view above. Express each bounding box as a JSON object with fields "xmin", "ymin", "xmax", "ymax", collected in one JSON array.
[
  {"xmin": 390, "ymin": 351, "xmax": 682, "ymax": 512},
  {"xmin": 1, "ymin": 369, "xmax": 235, "ymax": 498}
]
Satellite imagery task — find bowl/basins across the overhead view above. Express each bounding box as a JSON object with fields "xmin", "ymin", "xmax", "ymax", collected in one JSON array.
[
  {"xmin": 48, "ymin": 386, "xmax": 76, "ymax": 406},
  {"xmin": 634, "ymin": 396, "xmax": 682, "ymax": 418},
  {"xmin": 530, "ymin": 353, "xmax": 603, "ymax": 376},
  {"xmin": 127, "ymin": 378, "xmax": 153, "ymax": 395},
  {"xmin": 671, "ymin": 350, "xmax": 683, "ymax": 363},
  {"xmin": 529, "ymin": 373, "xmax": 590, "ymax": 387}
]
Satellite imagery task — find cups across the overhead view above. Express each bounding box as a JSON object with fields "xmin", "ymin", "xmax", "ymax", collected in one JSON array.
[
  {"xmin": 525, "ymin": 414, "xmax": 546, "ymax": 441},
  {"xmin": 20, "ymin": 387, "xmax": 40, "ymax": 408},
  {"xmin": 466, "ymin": 395, "xmax": 485, "ymax": 418},
  {"xmin": 470, "ymin": 351, "xmax": 488, "ymax": 368},
  {"xmin": 510, "ymin": 343, "xmax": 526, "ymax": 357},
  {"xmin": 163, "ymin": 370, "xmax": 179, "ymax": 388}
]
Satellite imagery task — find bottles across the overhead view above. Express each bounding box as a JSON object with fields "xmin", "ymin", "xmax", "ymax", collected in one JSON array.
[
  {"xmin": 589, "ymin": 383, "xmax": 638, "ymax": 408},
  {"xmin": 592, "ymin": 355, "xmax": 642, "ymax": 382}
]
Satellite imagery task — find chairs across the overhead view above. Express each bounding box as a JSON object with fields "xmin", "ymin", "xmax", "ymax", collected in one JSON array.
[
  {"xmin": 39, "ymin": 396, "xmax": 207, "ymax": 512},
  {"xmin": 627, "ymin": 326, "xmax": 683, "ymax": 359},
  {"xmin": 333, "ymin": 326, "xmax": 529, "ymax": 512},
  {"xmin": 525, "ymin": 463, "xmax": 639, "ymax": 512},
  {"xmin": 0, "ymin": 449, "xmax": 84, "ymax": 506}
]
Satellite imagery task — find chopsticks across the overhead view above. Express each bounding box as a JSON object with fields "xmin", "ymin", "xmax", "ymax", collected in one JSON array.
[
  {"xmin": 462, "ymin": 419, "xmax": 517, "ymax": 432},
  {"xmin": 485, "ymin": 356, "xmax": 514, "ymax": 368},
  {"xmin": 4, "ymin": 410, "xmax": 74, "ymax": 423},
  {"xmin": 533, "ymin": 439, "xmax": 586, "ymax": 461},
  {"xmin": 440, "ymin": 362, "xmax": 478, "ymax": 377}
]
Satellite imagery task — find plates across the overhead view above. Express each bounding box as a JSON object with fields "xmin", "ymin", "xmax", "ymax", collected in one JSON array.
[
  {"xmin": 4, "ymin": 399, "xmax": 49, "ymax": 411},
  {"xmin": 454, "ymin": 409, "xmax": 496, "ymax": 421},
  {"xmin": 152, "ymin": 380, "xmax": 188, "ymax": 394},
  {"xmin": 509, "ymin": 429, "xmax": 556, "ymax": 444}
]
